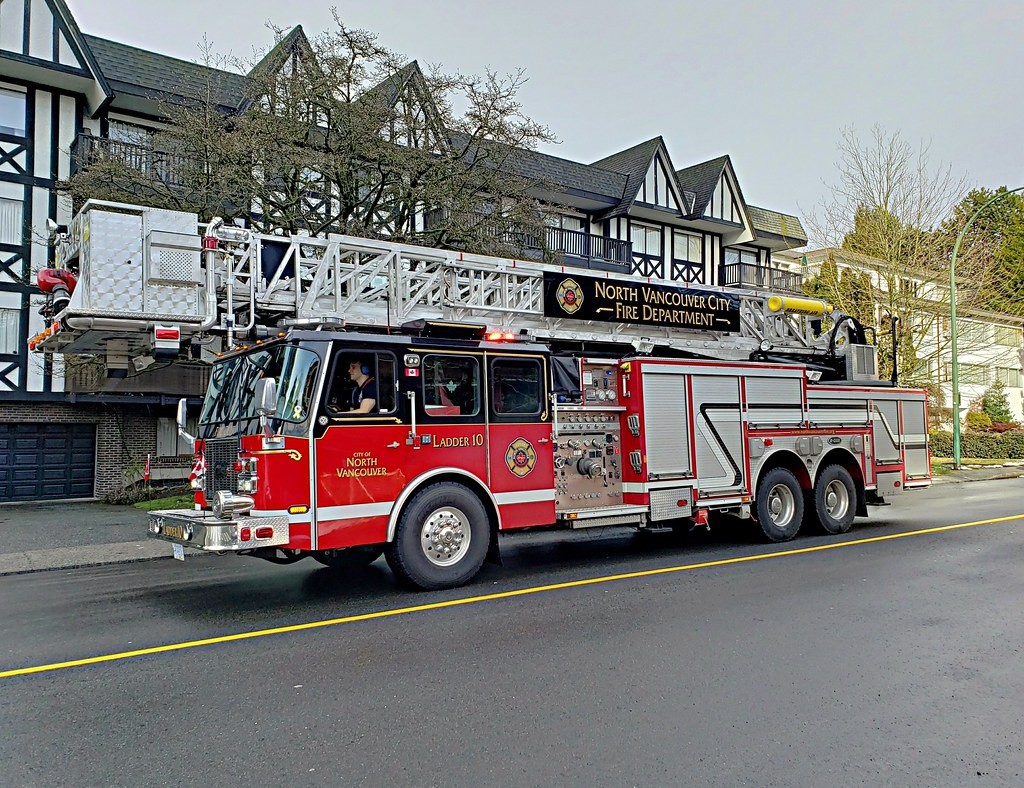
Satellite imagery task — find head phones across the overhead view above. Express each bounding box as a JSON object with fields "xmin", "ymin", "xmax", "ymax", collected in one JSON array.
[{"xmin": 360, "ymin": 353, "xmax": 370, "ymax": 375}]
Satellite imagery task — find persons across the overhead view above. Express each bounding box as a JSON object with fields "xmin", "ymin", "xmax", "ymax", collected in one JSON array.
[{"xmin": 334, "ymin": 357, "xmax": 379, "ymax": 413}]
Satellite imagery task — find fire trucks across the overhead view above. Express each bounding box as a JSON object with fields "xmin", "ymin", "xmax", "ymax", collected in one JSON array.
[{"xmin": 26, "ymin": 198, "xmax": 931, "ymax": 593}]
